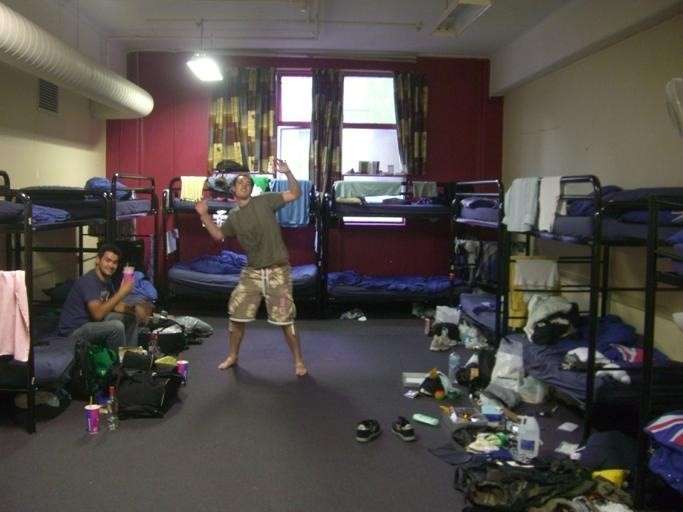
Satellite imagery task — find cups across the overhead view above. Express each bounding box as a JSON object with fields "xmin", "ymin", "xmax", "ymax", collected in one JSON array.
[
  {"xmin": 359, "ymin": 160, "xmax": 370, "ymax": 174},
  {"xmin": 122, "ymin": 267, "xmax": 135, "ymax": 282},
  {"xmin": 387, "ymin": 164, "xmax": 394, "ymax": 176},
  {"xmin": 424, "ymin": 317, "xmax": 432, "ymax": 337},
  {"xmin": 84, "ymin": 404, "xmax": 100, "ymax": 436},
  {"xmin": 178, "ymin": 360, "xmax": 188, "ymax": 385},
  {"xmin": 372, "ymin": 161, "xmax": 380, "ymax": 174}
]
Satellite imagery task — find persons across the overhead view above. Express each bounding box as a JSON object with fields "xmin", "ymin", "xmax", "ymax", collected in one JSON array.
[
  {"xmin": 57, "ymin": 243, "xmax": 149, "ymax": 353},
  {"xmin": 195, "ymin": 158, "xmax": 308, "ymax": 378}
]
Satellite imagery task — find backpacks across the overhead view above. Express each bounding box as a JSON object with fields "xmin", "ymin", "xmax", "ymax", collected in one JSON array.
[{"xmin": 70, "ymin": 339, "xmax": 117, "ymax": 400}]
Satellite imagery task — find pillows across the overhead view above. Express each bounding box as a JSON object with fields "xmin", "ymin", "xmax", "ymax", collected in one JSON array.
[
  {"xmin": 599, "ymin": 343, "xmax": 667, "ymax": 363},
  {"xmin": 460, "ymin": 196, "xmax": 495, "ymax": 208},
  {"xmin": 642, "ymin": 409, "xmax": 683, "ymax": 452}
]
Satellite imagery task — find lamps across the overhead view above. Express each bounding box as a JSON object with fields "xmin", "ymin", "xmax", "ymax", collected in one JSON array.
[{"xmin": 186, "ymin": 50, "xmax": 224, "ymax": 82}]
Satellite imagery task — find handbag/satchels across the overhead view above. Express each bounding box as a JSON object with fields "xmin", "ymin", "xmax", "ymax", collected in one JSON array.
[{"xmin": 104, "ymin": 353, "xmax": 180, "ymax": 419}]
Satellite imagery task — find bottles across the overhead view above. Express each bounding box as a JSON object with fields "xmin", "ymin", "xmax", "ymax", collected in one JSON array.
[
  {"xmin": 149, "ymin": 328, "xmax": 159, "ymax": 356},
  {"xmin": 449, "ymin": 265, "xmax": 455, "ymax": 280},
  {"xmin": 458, "ymin": 304, "xmax": 463, "ymax": 319},
  {"xmin": 105, "ymin": 386, "xmax": 121, "ymax": 430},
  {"xmin": 448, "ymin": 347, "xmax": 459, "ymax": 384}
]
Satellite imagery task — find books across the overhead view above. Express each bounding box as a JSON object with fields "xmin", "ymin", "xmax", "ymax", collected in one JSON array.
[{"xmin": 402, "ymin": 371, "xmax": 426, "ymax": 386}]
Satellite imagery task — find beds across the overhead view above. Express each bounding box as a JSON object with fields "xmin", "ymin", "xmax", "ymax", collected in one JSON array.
[
  {"xmin": 0, "ymin": 171, "xmax": 108, "ymax": 434},
  {"xmin": 634, "ymin": 193, "xmax": 683, "ymax": 511},
  {"xmin": 15, "ymin": 172, "xmax": 157, "ymax": 289},
  {"xmin": 321, "ymin": 180, "xmax": 474, "ymax": 316},
  {"xmin": 162, "ymin": 176, "xmax": 321, "ymax": 319},
  {"xmin": 502, "ymin": 175, "xmax": 683, "ymax": 418},
  {"xmin": 452, "ymin": 175, "xmax": 608, "ymax": 341}
]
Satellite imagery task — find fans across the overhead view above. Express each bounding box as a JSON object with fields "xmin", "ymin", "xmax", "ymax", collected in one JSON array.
[{"xmin": 665, "ymin": 77, "xmax": 683, "ymax": 141}]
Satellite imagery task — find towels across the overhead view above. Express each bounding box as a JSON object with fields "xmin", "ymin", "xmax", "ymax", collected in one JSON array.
[
  {"xmin": 0, "ymin": 270, "xmax": 31, "ymax": 362},
  {"xmin": 178, "ymin": 176, "xmax": 208, "ymax": 202},
  {"xmin": 270, "ymin": 178, "xmax": 315, "ymax": 229},
  {"xmin": 334, "ymin": 181, "xmax": 402, "ymax": 197}
]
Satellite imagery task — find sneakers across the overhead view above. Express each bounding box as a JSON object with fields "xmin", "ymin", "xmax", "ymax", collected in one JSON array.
[
  {"xmin": 355, "ymin": 419, "xmax": 382, "ymax": 443},
  {"xmin": 430, "ymin": 334, "xmax": 458, "ymax": 351},
  {"xmin": 391, "ymin": 416, "xmax": 416, "ymax": 442}
]
